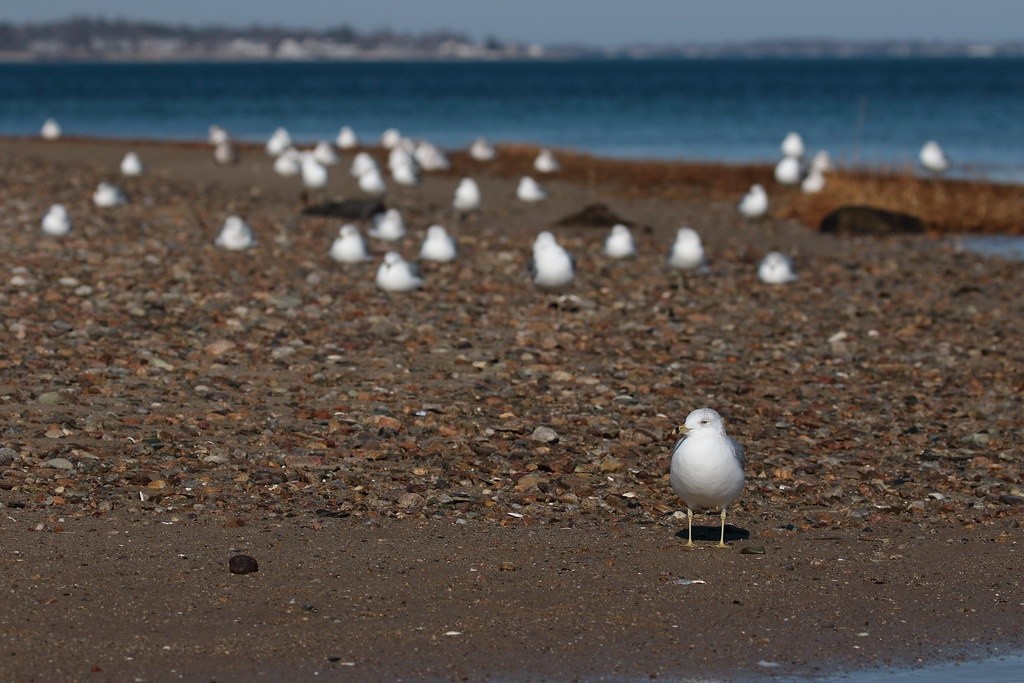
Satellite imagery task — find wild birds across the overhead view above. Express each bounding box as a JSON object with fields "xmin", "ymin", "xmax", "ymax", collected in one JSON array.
[
  {"xmin": 122, "ymin": 152, "xmax": 142, "ymax": 175},
  {"xmin": 215, "ymin": 215, "xmax": 258, "ymax": 251},
  {"xmin": 472, "ymin": 138, "xmax": 495, "ymax": 160},
  {"xmin": 516, "ymin": 175, "xmax": 551, "ymax": 203},
  {"xmin": 331, "ymin": 224, "xmax": 371, "ymax": 263},
  {"xmin": 93, "ymin": 181, "xmax": 127, "ymax": 207},
  {"xmin": 266, "ymin": 128, "xmax": 340, "ymax": 187},
  {"xmin": 603, "ymin": 224, "xmax": 637, "ymax": 258},
  {"xmin": 534, "ymin": 148, "xmax": 562, "ymax": 173},
  {"xmin": 670, "ymin": 407, "xmax": 744, "ymax": 550},
  {"xmin": 209, "ymin": 125, "xmax": 236, "ymax": 164},
  {"xmin": 350, "ymin": 152, "xmax": 387, "ymax": 192},
  {"xmin": 41, "ymin": 119, "xmax": 61, "ymax": 141},
  {"xmin": 528, "ymin": 231, "xmax": 577, "ymax": 288},
  {"xmin": 739, "ymin": 131, "xmax": 948, "ymax": 284},
  {"xmin": 666, "ymin": 227, "xmax": 705, "ymax": 269},
  {"xmin": 336, "ymin": 125, "xmax": 357, "ymax": 149},
  {"xmin": 376, "ymin": 224, "xmax": 456, "ymax": 291},
  {"xmin": 367, "ymin": 208, "xmax": 405, "ymax": 241},
  {"xmin": 381, "ymin": 128, "xmax": 450, "ymax": 186},
  {"xmin": 453, "ymin": 177, "xmax": 485, "ymax": 226},
  {"xmin": 41, "ymin": 204, "xmax": 72, "ymax": 237}
]
